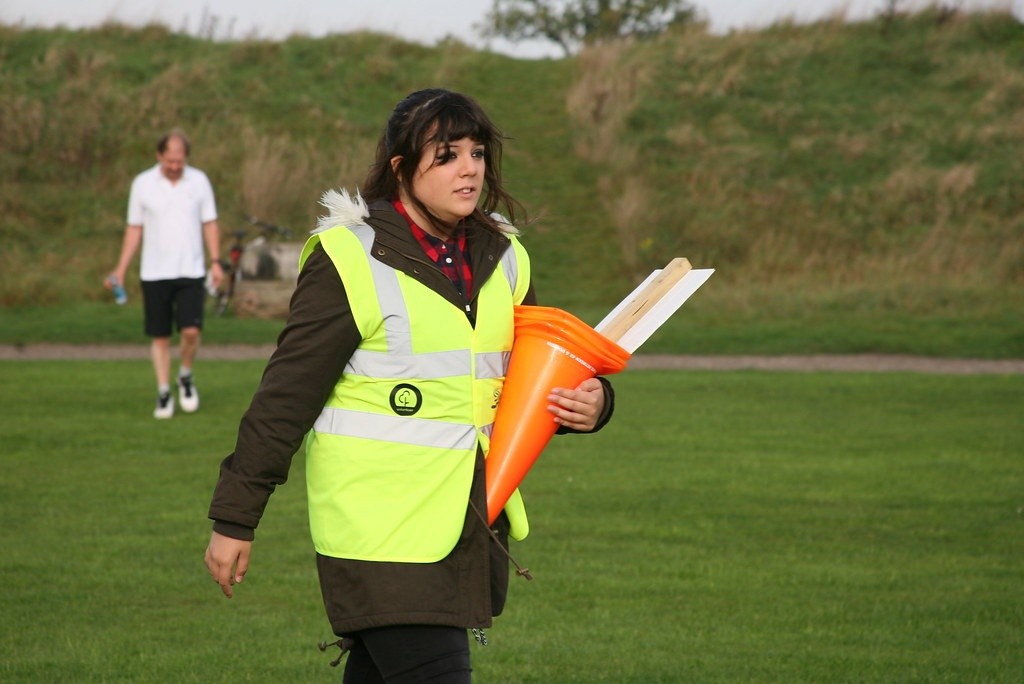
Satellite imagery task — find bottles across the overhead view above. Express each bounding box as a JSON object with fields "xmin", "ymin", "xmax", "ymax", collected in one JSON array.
[{"xmin": 108, "ymin": 276, "xmax": 128, "ymax": 304}]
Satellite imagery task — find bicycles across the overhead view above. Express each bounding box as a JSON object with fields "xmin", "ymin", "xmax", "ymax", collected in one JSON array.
[
  {"xmin": 243, "ymin": 217, "xmax": 288, "ymax": 284},
  {"xmin": 219, "ymin": 225, "xmax": 249, "ymax": 312}
]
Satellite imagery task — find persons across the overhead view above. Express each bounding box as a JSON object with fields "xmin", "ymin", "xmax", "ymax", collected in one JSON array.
[
  {"xmin": 103, "ymin": 133, "xmax": 224, "ymax": 419},
  {"xmin": 204, "ymin": 90, "xmax": 615, "ymax": 684}
]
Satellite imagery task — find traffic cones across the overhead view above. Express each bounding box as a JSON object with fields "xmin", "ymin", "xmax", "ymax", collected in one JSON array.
[{"xmin": 480, "ymin": 305, "xmax": 631, "ymax": 526}]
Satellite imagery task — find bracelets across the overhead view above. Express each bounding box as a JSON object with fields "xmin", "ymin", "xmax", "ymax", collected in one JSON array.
[{"xmin": 211, "ymin": 259, "xmax": 220, "ymax": 262}]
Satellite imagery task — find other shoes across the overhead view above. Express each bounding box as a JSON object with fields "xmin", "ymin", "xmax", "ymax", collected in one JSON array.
[
  {"xmin": 152, "ymin": 390, "xmax": 177, "ymax": 419},
  {"xmin": 177, "ymin": 372, "xmax": 200, "ymax": 413}
]
